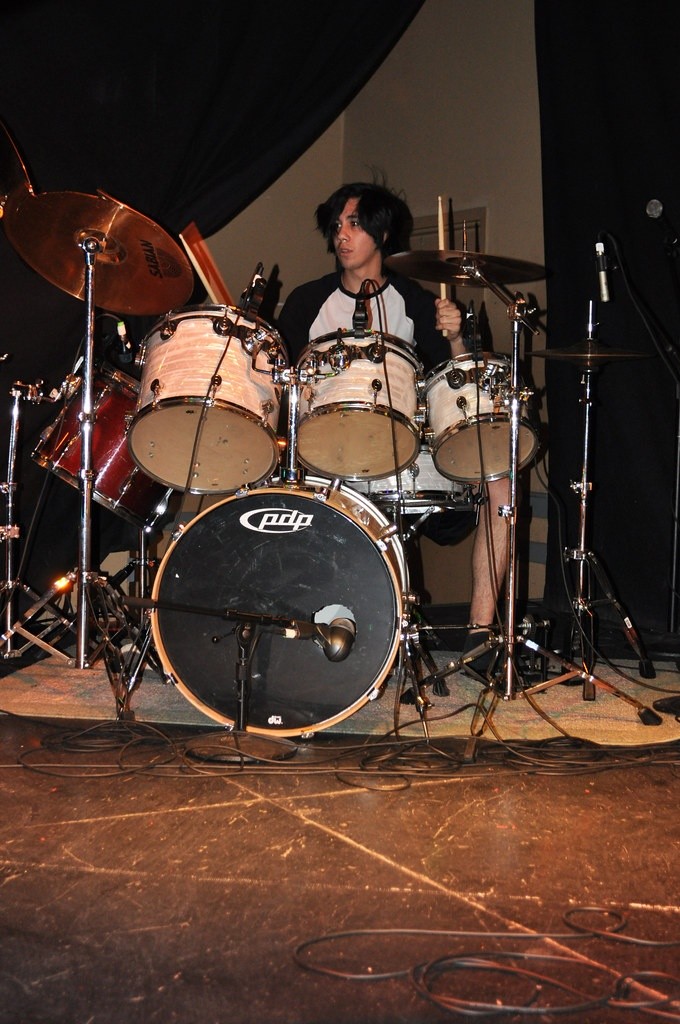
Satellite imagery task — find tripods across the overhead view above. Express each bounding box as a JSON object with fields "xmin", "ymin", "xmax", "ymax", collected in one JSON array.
[
  {"xmin": 401, "ymin": 256, "xmax": 664, "ymax": 765},
  {"xmin": 0, "ymin": 232, "xmax": 174, "ymax": 689}
]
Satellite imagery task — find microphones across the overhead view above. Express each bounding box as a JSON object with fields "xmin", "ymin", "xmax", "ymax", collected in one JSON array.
[
  {"xmin": 352, "ymin": 282, "xmax": 368, "ymax": 328},
  {"xmin": 462, "ymin": 302, "xmax": 479, "ymax": 349},
  {"xmin": 239, "ymin": 267, "xmax": 264, "ymax": 310},
  {"xmin": 596, "ymin": 238, "xmax": 609, "ymax": 302},
  {"xmin": 117, "ymin": 320, "xmax": 132, "ymax": 363},
  {"xmin": 283, "ymin": 618, "xmax": 356, "ymax": 662}
]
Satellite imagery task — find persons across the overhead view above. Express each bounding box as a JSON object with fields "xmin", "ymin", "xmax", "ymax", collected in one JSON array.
[{"xmin": 277, "ymin": 162, "xmax": 523, "ymax": 699}]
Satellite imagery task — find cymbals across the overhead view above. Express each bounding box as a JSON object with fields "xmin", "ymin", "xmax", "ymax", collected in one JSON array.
[
  {"xmin": 523, "ymin": 340, "xmax": 648, "ymax": 367},
  {"xmin": 3, "ymin": 181, "xmax": 194, "ymax": 315},
  {"xmin": 383, "ymin": 249, "xmax": 545, "ymax": 288}
]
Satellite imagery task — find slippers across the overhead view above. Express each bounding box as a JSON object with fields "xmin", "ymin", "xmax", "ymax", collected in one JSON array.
[{"xmin": 461, "ymin": 663, "xmax": 517, "ymax": 695}]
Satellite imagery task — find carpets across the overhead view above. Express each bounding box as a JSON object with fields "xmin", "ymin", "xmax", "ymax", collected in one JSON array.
[{"xmin": 1, "ymin": 645, "xmax": 680, "ymax": 746}]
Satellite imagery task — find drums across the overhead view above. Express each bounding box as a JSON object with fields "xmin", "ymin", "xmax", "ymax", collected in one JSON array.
[
  {"xmin": 150, "ymin": 476, "xmax": 412, "ymax": 738},
  {"xmin": 423, "ymin": 350, "xmax": 539, "ymax": 483},
  {"xmin": 293, "ymin": 329, "xmax": 422, "ymax": 482},
  {"xmin": 127, "ymin": 302, "xmax": 289, "ymax": 496},
  {"xmin": 31, "ymin": 361, "xmax": 175, "ymax": 535},
  {"xmin": 342, "ymin": 443, "xmax": 475, "ymax": 515}
]
